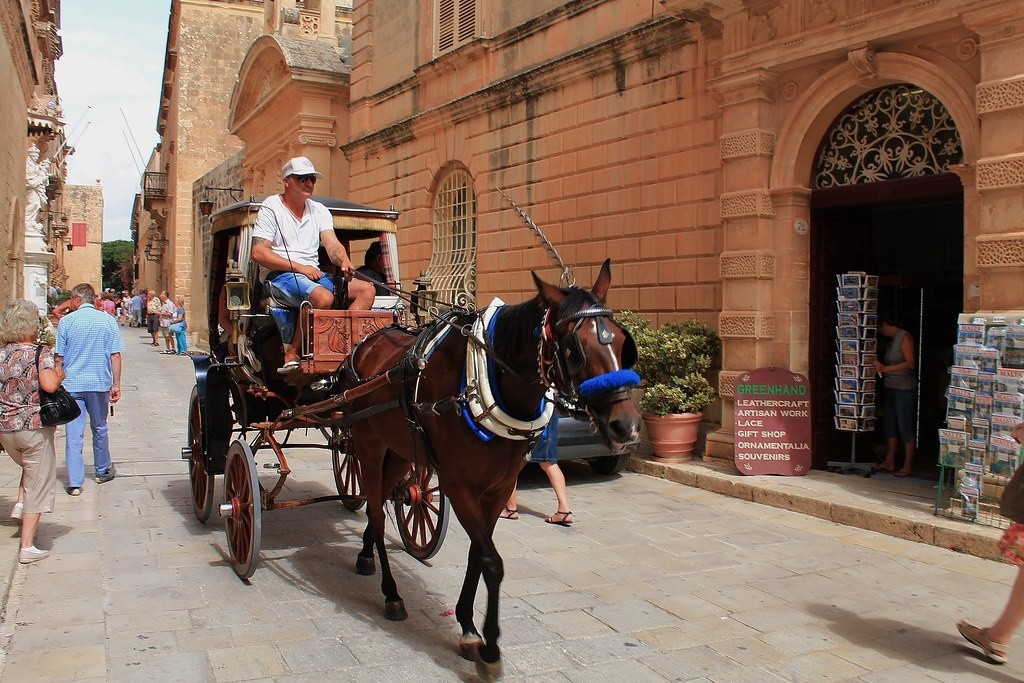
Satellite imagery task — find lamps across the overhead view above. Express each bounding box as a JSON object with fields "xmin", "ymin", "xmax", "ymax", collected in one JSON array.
[
  {"xmin": 52, "ymin": 211, "xmax": 68, "ymax": 222},
  {"xmin": 199, "ymin": 186, "xmax": 244, "ymax": 217},
  {"xmin": 63, "ymin": 238, "xmax": 73, "ymax": 251}
]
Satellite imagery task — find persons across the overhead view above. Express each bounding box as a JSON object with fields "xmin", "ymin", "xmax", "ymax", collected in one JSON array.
[
  {"xmin": 53, "ymin": 283, "xmax": 186, "ymax": 496},
  {"xmin": 875, "ymin": 313, "xmax": 917, "ymax": 475},
  {"xmin": 24, "ymin": 145, "xmax": 51, "ymax": 233},
  {"xmin": 250, "ymin": 156, "xmax": 391, "ymax": 374},
  {"xmin": 1, "ymin": 299, "xmax": 66, "ymax": 563},
  {"xmin": 498, "ymin": 411, "xmax": 574, "ymax": 524},
  {"xmin": 957, "ymin": 424, "xmax": 1024, "ymax": 663}
]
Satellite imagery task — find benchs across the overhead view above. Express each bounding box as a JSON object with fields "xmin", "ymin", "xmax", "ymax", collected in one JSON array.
[{"xmin": 258, "ymin": 282, "xmax": 405, "ymax": 313}]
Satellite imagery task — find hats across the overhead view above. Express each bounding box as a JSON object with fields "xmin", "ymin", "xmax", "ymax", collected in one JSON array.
[{"xmin": 281, "ymin": 157, "xmax": 323, "ymax": 181}]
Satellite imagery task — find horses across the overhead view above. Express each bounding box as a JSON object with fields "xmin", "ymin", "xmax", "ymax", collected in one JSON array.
[{"xmin": 339, "ymin": 259, "xmax": 642, "ymax": 683}]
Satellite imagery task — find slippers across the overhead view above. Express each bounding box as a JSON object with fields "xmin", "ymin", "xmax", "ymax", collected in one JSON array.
[
  {"xmin": 876, "ymin": 463, "xmax": 913, "ymax": 477},
  {"xmin": 276, "ymin": 361, "xmax": 299, "ymax": 375}
]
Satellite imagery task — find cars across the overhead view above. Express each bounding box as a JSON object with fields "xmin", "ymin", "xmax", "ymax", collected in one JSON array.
[{"xmin": 521, "ymin": 393, "xmax": 632, "ymax": 476}]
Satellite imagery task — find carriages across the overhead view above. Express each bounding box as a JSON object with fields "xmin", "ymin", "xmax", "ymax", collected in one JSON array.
[{"xmin": 182, "ymin": 192, "xmax": 643, "ymax": 683}]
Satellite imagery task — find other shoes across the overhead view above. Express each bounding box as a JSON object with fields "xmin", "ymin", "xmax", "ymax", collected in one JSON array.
[
  {"xmin": 19, "ymin": 549, "xmax": 49, "ymax": 564},
  {"xmin": 11, "ymin": 506, "xmax": 23, "ymax": 524},
  {"xmin": 96, "ymin": 467, "xmax": 115, "ymax": 484},
  {"xmin": 66, "ymin": 486, "xmax": 80, "ymax": 495},
  {"xmin": 151, "ymin": 342, "xmax": 159, "ymax": 347},
  {"xmin": 160, "ymin": 349, "xmax": 176, "ymax": 354}
]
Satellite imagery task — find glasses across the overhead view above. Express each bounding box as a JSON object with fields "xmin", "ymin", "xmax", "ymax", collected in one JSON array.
[{"xmin": 295, "ymin": 175, "xmax": 316, "ymax": 184}]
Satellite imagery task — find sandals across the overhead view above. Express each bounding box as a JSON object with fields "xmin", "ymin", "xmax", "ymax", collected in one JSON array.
[
  {"xmin": 956, "ymin": 619, "xmax": 1007, "ymax": 664},
  {"xmin": 545, "ymin": 510, "xmax": 574, "ymax": 526},
  {"xmin": 499, "ymin": 505, "xmax": 519, "ymax": 520}
]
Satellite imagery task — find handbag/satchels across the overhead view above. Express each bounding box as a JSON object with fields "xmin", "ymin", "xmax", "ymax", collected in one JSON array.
[
  {"xmin": 35, "ymin": 345, "xmax": 81, "ymax": 427},
  {"xmin": 171, "ymin": 321, "xmax": 185, "ymax": 334}
]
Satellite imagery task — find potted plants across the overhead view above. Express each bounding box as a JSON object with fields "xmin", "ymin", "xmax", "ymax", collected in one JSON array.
[{"xmin": 638, "ymin": 371, "xmax": 716, "ymax": 463}]
